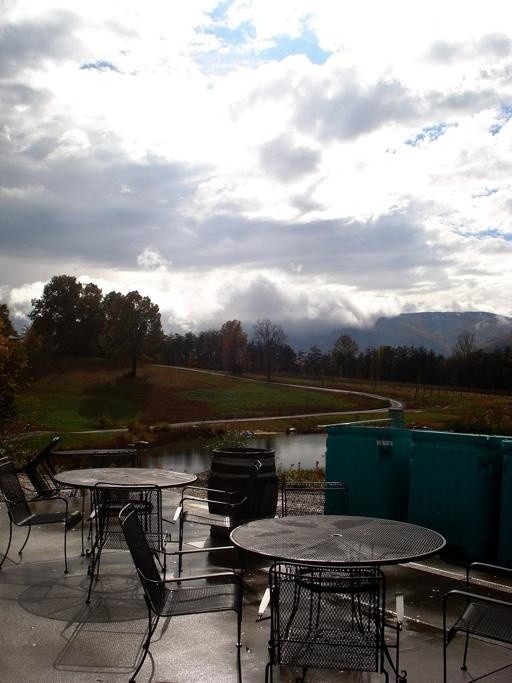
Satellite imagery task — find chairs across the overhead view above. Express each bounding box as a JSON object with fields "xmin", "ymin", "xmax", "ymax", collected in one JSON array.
[
  {"xmin": 442, "ymin": 561, "xmax": 512, "ymax": 683},
  {"xmin": 85, "ymin": 482, "xmax": 173, "ymax": 604},
  {"xmin": 178, "ymin": 459, "xmax": 262, "ymax": 572},
  {"xmin": 0, "ymin": 456, "xmax": 86, "ymax": 575},
  {"xmin": 22, "ymin": 433, "xmax": 70, "ymax": 498},
  {"xmin": 280, "ymin": 480, "xmax": 348, "ymax": 515},
  {"xmin": 118, "ymin": 503, "xmax": 249, "ymax": 683},
  {"xmin": 265, "ymin": 561, "xmax": 408, "ymax": 682}
]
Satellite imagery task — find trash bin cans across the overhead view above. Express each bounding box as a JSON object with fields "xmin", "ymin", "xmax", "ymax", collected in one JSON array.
[{"xmin": 204, "ymin": 445, "xmax": 278, "ymax": 539}]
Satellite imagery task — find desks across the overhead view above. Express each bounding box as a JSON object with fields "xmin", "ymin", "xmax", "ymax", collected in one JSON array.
[
  {"xmin": 229, "ymin": 515, "xmax": 449, "ymax": 667},
  {"xmin": 52, "ymin": 467, "xmax": 198, "ymax": 574}
]
